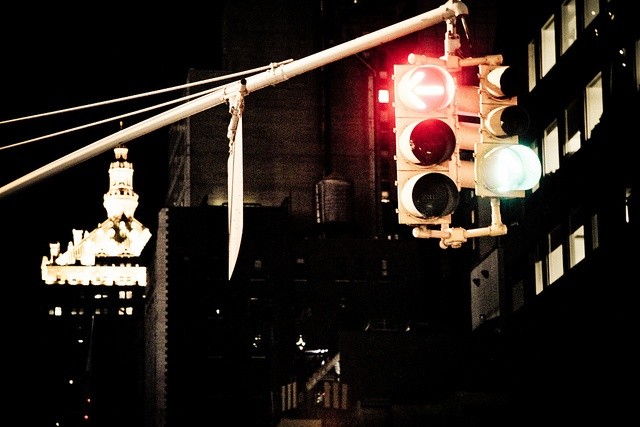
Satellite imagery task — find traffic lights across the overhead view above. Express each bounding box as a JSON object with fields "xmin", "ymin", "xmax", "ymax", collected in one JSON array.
[
  {"xmin": 475, "ymin": 63, "xmax": 541, "ymax": 200},
  {"xmin": 393, "ymin": 64, "xmax": 461, "ymax": 223}
]
